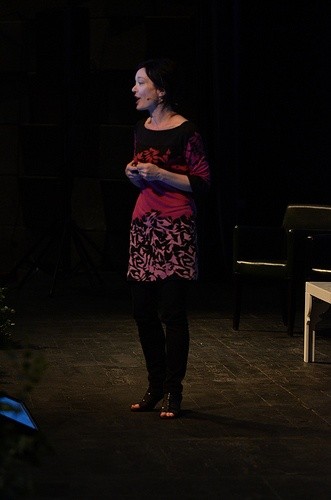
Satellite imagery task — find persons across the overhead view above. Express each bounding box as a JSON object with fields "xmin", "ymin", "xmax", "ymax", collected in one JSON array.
[{"xmin": 125, "ymin": 61, "xmax": 212, "ymax": 420}]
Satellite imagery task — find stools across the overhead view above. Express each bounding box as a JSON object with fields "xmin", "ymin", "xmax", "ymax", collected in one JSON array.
[{"xmin": 303, "ymin": 281, "xmax": 331, "ymax": 362}]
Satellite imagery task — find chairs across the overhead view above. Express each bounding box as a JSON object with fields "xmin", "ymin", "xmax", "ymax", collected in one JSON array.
[{"xmin": 233, "ymin": 225, "xmax": 294, "ymax": 330}]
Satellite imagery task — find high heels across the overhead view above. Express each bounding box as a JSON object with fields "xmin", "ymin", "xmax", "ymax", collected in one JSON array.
[
  {"xmin": 160, "ymin": 384, "xmax": 183, "ymax": 418},
  {"xmin": 129, "ymin": 381, "xmax": 167, "ymax": 413}
]
